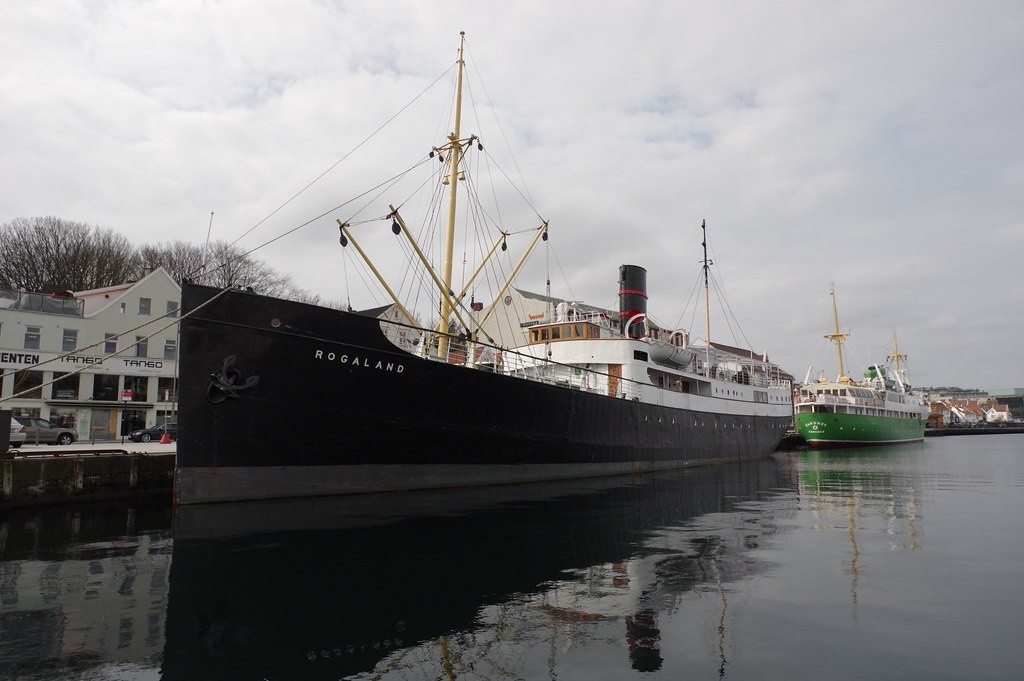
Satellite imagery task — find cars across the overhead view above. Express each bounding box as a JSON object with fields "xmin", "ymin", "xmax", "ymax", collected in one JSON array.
[
  {"xmin": 14, "ymin": 418, "xmax": 79, "ymax": 445},
  {"xmin": 926, "ymin": 423, "xmax": 935, "ymax": 428},
  {"xmin": 948, "ymin": 422, "xmax": 966, "ymax": 428},
  {"xmin": 9, "ymin": 416, "xmax": 27, "ymax": 448},
  {"xmin": 128, "ymin": 421, "xmax": 178, "ymax": 443}
]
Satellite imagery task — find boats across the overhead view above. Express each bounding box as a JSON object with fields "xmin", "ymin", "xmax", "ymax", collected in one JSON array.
[
  {"xmin": 158, "ymin": 456, "xmax": 799, "ymax": 681},
  {"xmin": 794, "ymin": 283, "xmax": 933, "ymax": 448},
  {"xmin": 169, "ymin": 30, "xmax": 794, "ymax": 505},
  {"xmin": 647, "ymin": 338, "xmax": 697, "ymax": 368}
]
